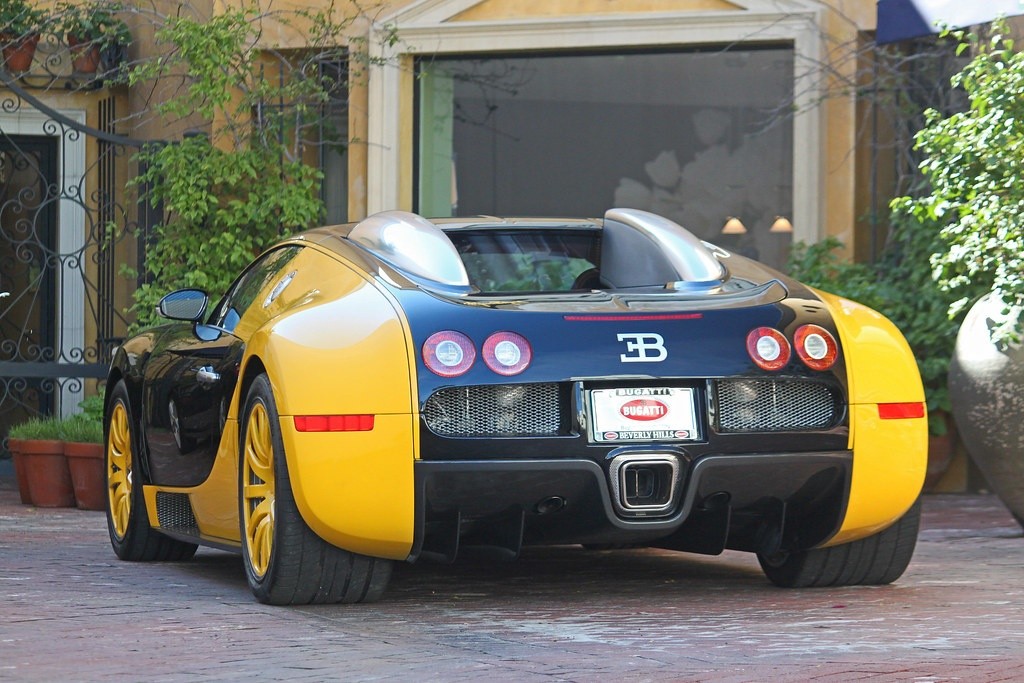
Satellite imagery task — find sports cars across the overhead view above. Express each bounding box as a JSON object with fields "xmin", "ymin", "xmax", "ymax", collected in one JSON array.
[{"xmin": 102, "ymin": 209, "xmax": 929, "ymax": 607}]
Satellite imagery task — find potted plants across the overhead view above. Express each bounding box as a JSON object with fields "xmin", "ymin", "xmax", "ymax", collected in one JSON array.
[
  {"xmin": 0, "ymin": 0, "xmax": 130, "ymax": 76},
  {"xmin": 890, "ymin": 15, "xmax": 1024, "ymax": 533},
  {"xmin": 6, "ymin": 414, "xmax": 107, "ymax": 510}
]
